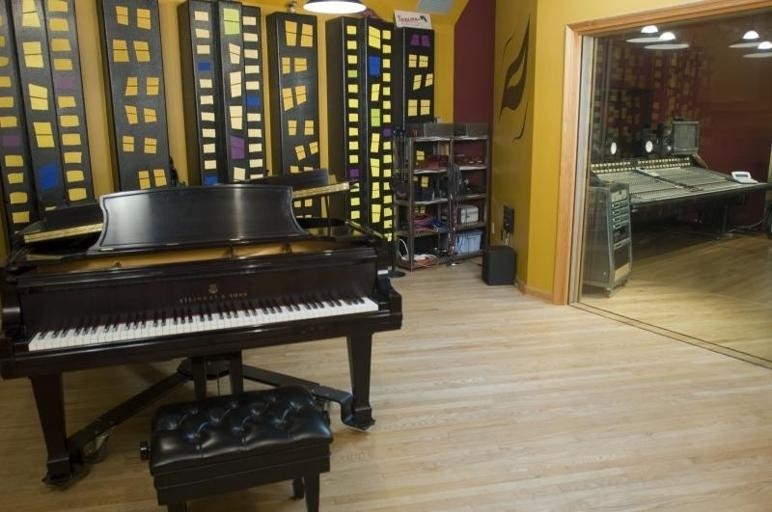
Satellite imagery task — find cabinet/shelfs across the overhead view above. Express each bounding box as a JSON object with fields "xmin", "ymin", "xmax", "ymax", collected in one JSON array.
[{"xmin": 392, "ymin": 134, "xmax": 492, "ymax": 273}]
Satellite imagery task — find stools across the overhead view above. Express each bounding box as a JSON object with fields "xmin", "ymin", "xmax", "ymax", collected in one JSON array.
[{"xmin": 144, "ymin": 383, "xmax": 332, "ymax": 510}]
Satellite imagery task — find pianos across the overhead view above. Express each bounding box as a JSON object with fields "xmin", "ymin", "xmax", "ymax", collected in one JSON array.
[{"xmin": 0, "ymin": 184, "xmax": 402, "ymax": 487}]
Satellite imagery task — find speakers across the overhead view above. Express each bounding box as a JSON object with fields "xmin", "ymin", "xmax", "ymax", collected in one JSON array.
[{"xmin": 482, "ymin": 246, "xmax": 517, "ymax": 285}]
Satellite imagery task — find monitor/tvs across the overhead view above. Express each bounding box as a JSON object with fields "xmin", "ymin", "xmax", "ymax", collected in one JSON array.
[{"xmin": 672, "ymin": 120, "xmax": 700, "ymax": 155}]
